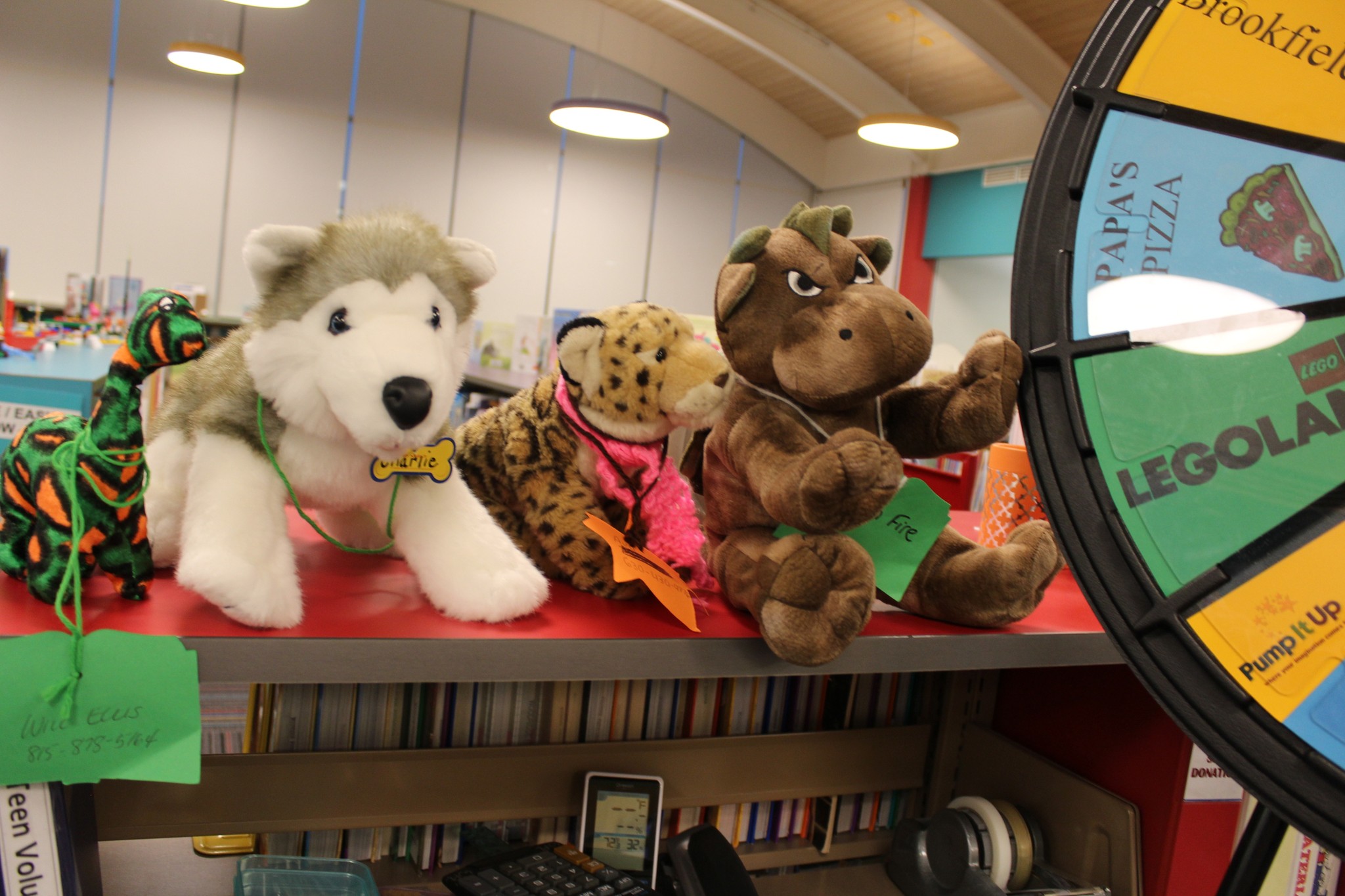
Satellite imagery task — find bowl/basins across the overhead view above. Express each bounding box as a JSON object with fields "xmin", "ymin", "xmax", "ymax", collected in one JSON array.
[{"xmin": 232, "ymin": 853, "xmax": 378, "ymax": 896}]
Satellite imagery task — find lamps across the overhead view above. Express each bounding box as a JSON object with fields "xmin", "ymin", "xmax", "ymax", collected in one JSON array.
[
  {"xmin": 164, "ymin": 41, "xmax": 246, "ymax": 76},
  {"xmin": 858, "ymin": 22, "xmax": 959, "ymax": 151},
  {"xmin": 548, "ymin": 96, "xmax": 671, "ymax": 141}
]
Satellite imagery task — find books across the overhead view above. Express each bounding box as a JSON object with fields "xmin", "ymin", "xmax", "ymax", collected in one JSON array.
[{"xmin": 243, "ymin": 684, "xmax": 1001, "ymax": 870}]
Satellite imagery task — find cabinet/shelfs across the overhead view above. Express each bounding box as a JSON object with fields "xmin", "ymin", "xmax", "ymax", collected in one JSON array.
[{"xmin": 0, "ymin": 541, "xmax": 1198, "ymax": 896}]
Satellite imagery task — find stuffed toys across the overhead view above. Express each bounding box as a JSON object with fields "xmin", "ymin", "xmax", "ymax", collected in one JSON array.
[
  {"xmin": 138, "ymin": 210, "xmax": 550, "ymax": 628},
  {"xmin": 697, "ymin": 201, "xmax": 1059, "ymax": 667},
  {"xmin": 452, "ymin": 300, "xmax": 732, "ymax": 601}
]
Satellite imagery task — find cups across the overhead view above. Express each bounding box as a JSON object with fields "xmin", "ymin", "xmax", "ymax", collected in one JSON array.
[{"xmin": 977, "ymin": 442, "xmax": 1047, "ymax": 547}]
